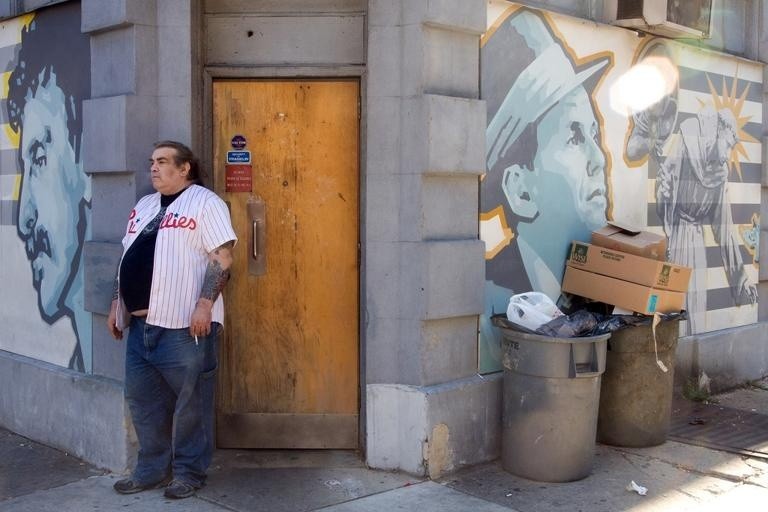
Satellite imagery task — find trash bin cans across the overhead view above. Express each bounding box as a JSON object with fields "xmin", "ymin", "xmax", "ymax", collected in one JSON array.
[
  {"xmin": 490, "ymin": 314, "xmax": 611, "ymax": 484},
  {"xmin": 596, "ymin": 314, "xmax": 687, "ymax": 446}
]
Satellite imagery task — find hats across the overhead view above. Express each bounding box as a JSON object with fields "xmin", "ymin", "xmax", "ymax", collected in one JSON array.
[{"xmin": 480, "ymin": 7, "xmax": 611, "ymax": 216}]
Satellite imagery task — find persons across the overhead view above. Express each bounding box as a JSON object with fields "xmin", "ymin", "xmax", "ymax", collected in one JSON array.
[
  {"xmin": 9, "ymin": 7, "xmax": 94, "ymax": 372},
  {"xmin": 105, "ymin": 139, "xmax": 240, "ymax": 499},
  {"xmin": 654, "ymin": 104, "xmax": 758, "ymax": 334},
  {"xmin": 480, "ymin": 5, "xmax": 614, "ymax": 305},
  {"xmin": 625, "ymin": 95, "xmax": 677, "ymax": 163}
]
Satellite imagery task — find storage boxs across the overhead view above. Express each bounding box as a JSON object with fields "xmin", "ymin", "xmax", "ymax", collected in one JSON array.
[{"xmin": 559, "ymin": 220, "xmax": 691, "ymax": 315}]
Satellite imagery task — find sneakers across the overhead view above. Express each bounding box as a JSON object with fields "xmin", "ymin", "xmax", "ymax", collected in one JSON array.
[
  {"xmin": 165, "ymin": 479, "xmax": 196, "ymax": 499},
  {"xmin": 114, "ymin": 478, "xmax": 171, "ymax": 493}
]
